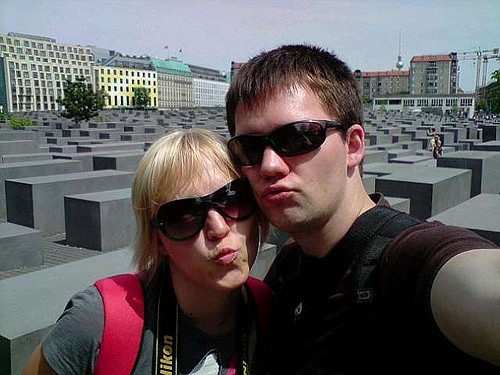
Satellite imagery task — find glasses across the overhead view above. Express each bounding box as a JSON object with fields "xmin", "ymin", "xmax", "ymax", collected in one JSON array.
[
  {"xmin": 147, "ymin": 176, "xmax": 259, "ymax": 242},
  {"xmin": 226, "ymin": 120, "xmax": 347, "ymax": 167}
]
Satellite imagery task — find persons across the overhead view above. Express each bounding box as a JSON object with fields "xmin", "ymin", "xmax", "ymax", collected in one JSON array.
[
  {"xmin": 430, "ymin": 135, "xmax": 439, "ymax": 150},
  {"xmin": 18, "ymin": 129, "xmax": 280, "ymax": 375},
  {"xmin": 434, "ymin": 139, "xmax": 443, "ymax": 158},
  {"xmin": 225, "ymin": 44, "xmax": 499, "ymax": 375}
]
